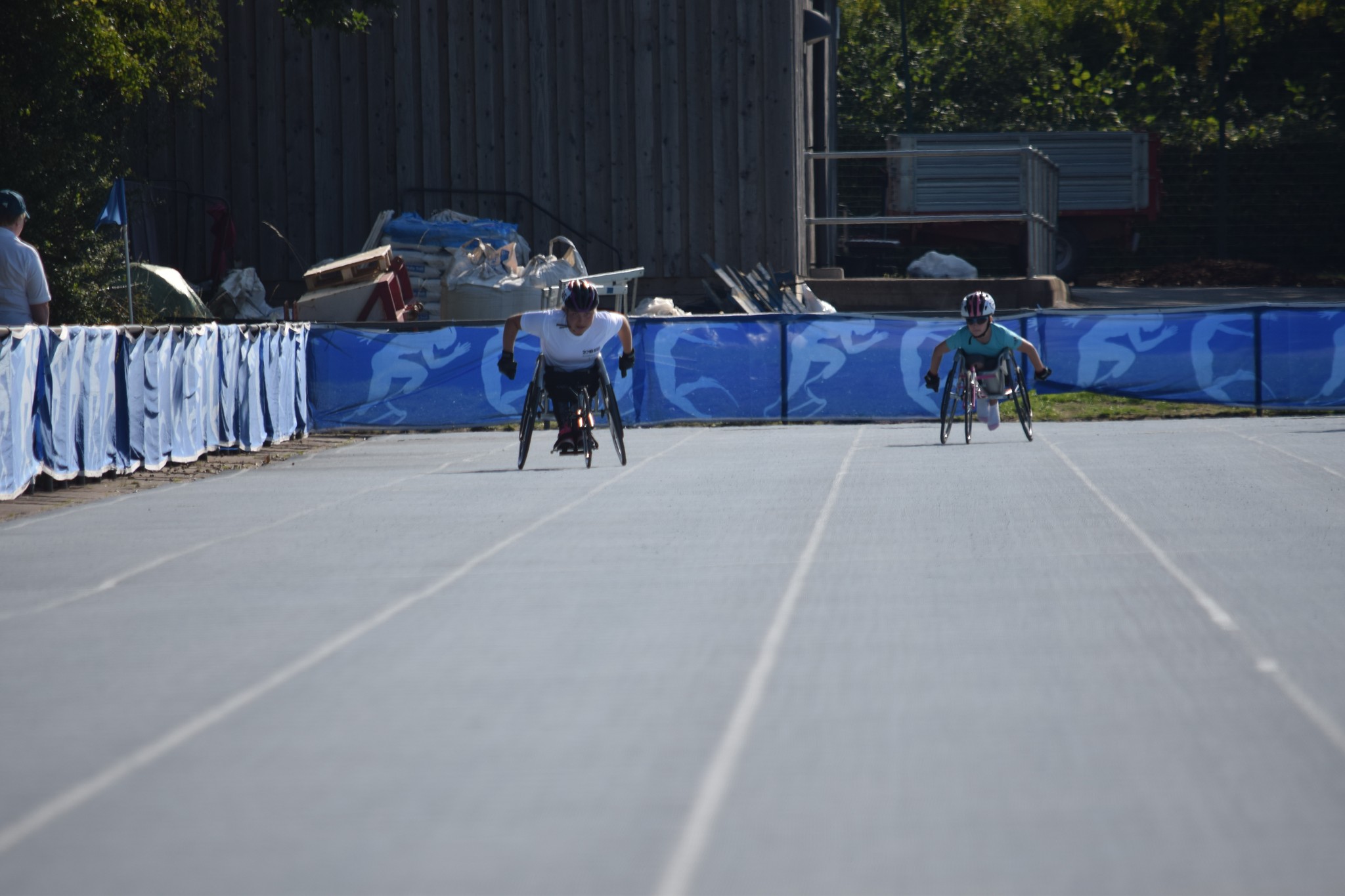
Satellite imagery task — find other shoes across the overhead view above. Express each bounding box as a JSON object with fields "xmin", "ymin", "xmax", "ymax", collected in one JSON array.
[
  {"xmin": 553, "ymin": 423, "xmax": 578, "ymax": 450},
  {"xmin": 577, "ymin": 427, "xmax": 599, "ymax": 450}
]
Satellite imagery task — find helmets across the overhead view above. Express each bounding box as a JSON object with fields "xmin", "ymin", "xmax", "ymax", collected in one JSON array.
[
  {"xmin": 562, "ymin": 279, "xmax": 600, "ymax": 312},
  {"xmin": 961, "ymin": 291, "xmax": 996, "ymax": 317}
]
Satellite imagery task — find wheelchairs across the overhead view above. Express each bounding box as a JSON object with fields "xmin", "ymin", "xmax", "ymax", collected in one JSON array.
[
  {"xmin": 940, "ymin": 346, "xmax": 1034, "ymax": 445},
  {"xmin": 517, "ymin": 351, "xmax": 627, "ymax": 468}
]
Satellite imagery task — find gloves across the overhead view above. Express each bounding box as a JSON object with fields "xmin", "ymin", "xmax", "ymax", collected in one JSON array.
[
  {"xmin": 924, "ymin": 369, "xmax": 940, "ymax": 392},
  {"xmin": 618, "ymin": 348, "xmax": 635, "ymax": 379},
  {"xmin": 1034, "ymin": 366, "xmax": 1052, "ymax": 382},
  {"xmin": 497, "ymin": 350, "xmax": 517, "ymax": 380}
]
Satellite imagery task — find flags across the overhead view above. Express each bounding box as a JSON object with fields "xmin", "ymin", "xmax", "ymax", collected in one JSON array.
[{"xmin": 95, "ymin": 185, "xmax": 121, "ymax": 233}]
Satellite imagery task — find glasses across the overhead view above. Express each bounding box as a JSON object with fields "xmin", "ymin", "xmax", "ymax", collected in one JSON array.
[{"xmin": 966, "ymin": 316, "xmax": 987, "ymax": 325}]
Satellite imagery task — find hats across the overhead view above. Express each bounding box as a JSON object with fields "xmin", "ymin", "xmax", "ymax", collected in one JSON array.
[{"xmin": 0, "ymin": 190, "xmax": 30, "ymax": 219}]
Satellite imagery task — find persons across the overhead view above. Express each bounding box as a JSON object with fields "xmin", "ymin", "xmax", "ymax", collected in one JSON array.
[
  {"xmin": 497, "ymin": 278, "xmax": 635, "ymax": 452},
  {"xmin": 925, "ymin": 291, "xmax": 1052, "ymax": 431},
  {"xmin": 0, "ymin": 187, "xmax": 52, "ymax": 327}
]
